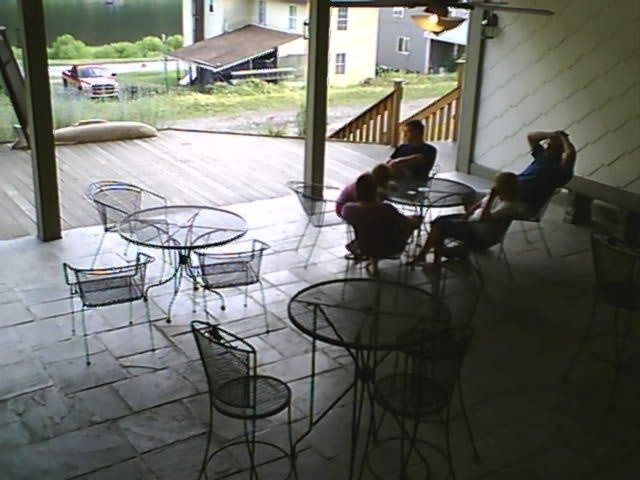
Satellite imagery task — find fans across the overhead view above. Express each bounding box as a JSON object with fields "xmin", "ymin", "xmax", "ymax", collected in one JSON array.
[{"xmin": 329, "ymin": 1, "xmax": 555, "ymax": 17}]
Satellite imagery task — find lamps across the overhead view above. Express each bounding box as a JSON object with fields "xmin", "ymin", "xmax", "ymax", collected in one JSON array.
[
  {"xmin": 410, "ymin": 5, "xmax": 465, "ymax": 36},
  {"xmin": 480, "ymin": 12, "xmax": 498, "ymax": 43}
]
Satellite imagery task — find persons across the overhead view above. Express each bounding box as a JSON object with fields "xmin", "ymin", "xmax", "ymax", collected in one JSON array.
[
  {"xmin": 342, "ymin": 173, "xmax": 422, "ymax": 257},
  {"xmin": 335, "ymin": 163, "xmax": 399, "ymax": 257},
  {"xmin": 386, "ymin": 119, "xmax": 437, "ymax": 182},
  {"xmin": 464, "ymin": 130, "xmax": 576, "ymax": 219},
  {"xmin": 405, "ymin": 172, "xmax": 519, "ymax": 266}
]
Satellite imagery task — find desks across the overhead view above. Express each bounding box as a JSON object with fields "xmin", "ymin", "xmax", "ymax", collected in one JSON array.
[
  {"xmin": 116, "ymin": 205, "xmax": 241, "ymax": 322},
  {"xmin": 288, "ymin": 277, "xmax": 454, "ymax": 479}
]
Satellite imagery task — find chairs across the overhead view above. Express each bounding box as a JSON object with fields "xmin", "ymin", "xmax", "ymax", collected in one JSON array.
[
  {"xmin": 285, "ymin": 168, "xmax": 557, "ymax": 297},
  {"xmin": 190, "ymin": 321, "xmax": 299, "ymax": 478},
  {"xmin": 191, "ymin": 240, "xmax": 270, "ymax": 341},
  {"xmin": 90, "ymin": 181, "xmax": 169, "ymax": 286},
  {"xmin": 356, "ymin": 325, "xmax": 482, "ymax": 479},
  {"xmin": 561, "ymin": 230, "xmax": 640, "ymax": 413},
  {"xmin": 61, "ymin": 252, "xmax": 154, "ymax": 365}
]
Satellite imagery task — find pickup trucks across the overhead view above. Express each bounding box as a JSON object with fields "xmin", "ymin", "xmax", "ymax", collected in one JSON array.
[{"xmin": 61, "ymin": 64, "xmax": 120, "ymax": 100}]
